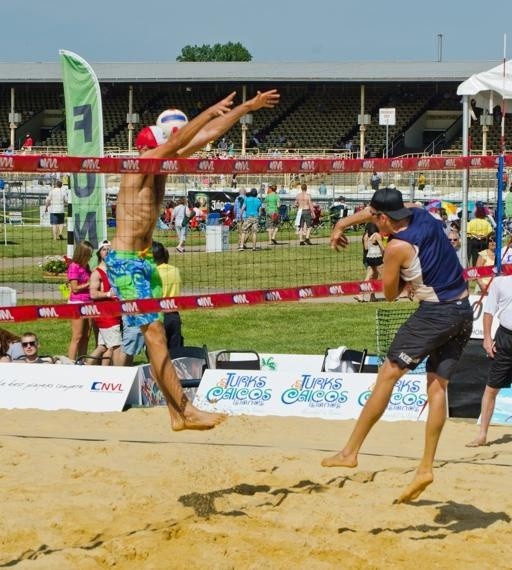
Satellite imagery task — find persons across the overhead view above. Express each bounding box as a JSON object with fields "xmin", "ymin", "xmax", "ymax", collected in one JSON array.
[
  {"xmin": 45, "ymin": 181, "xmax": 68, "ymax": 241},
  {"xmin": 320, "ymin": 188, "xmax": 473, "ymax": 502},
  {"xmin": 103, "ymin": 88, "xmax": 280, "ymax": 431},
  {"xmin": 204, "ymin": 130, "xmax": 293, "ymax": 156},
  {"xmin": 465, "ymin": 276, "xmax": 512, "ymax": 447},
  {"xmin": 0, "ymin": 327, "xmax": 22, "ymax": 362},
  {"xmin": 335, "ymin": 137, "xmax": 354, "ymax": 152},
  {"xmin": 22, "ymin": 133, "xmax": 33, "ymax": 151},
  {"xmin": 12, "ymin": 332, "xmax": 51, "ymax": 364}
]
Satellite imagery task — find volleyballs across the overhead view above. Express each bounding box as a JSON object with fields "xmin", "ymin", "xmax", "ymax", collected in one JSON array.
[{"xmin": 157, "ymin": 109, "xmax": 188, "ymax": 142}]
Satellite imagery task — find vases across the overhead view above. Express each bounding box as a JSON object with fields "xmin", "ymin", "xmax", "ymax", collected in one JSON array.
[{"xmin": 41, "ymin": 270, "xmax": 70, "ymax": 281}]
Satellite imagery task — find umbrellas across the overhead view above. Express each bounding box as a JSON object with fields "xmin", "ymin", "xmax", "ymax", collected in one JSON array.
[{"xmin": 456, "ymin": 58, "xmax": 512, "ymax": 114}]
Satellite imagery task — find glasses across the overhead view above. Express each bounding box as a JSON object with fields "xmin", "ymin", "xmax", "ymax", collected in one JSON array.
[
  {"xmin": 448, "ymin": 239, "xmax": 459, "ymax": 242},
  {"xmin": 22, "ymin": 341, "xmax": 36, "ymax": 348}
]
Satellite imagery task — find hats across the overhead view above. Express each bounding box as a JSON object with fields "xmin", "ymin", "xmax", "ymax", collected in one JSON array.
[
  {"xmin": 134, "ymin": 125, "xmax": 169, "ymax": 148},
  {"xmin": 370, "ymin": 187, "xmax": 414, "ymax": 223},
  {"xmin": 96, "ymin": 239, "xmax": 113, "ymax": 252}
]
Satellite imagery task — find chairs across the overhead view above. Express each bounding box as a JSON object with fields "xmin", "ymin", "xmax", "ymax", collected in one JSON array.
[
  {"xmin": 365, "ymin": 82, "xmax": 427, "ymax": 158},
  {"xmin": 247, "ymin": 82, "xmax": 360, "ymax": 157},
  {"xmin": 2, "ymin": 82, "xmax": 67, "ymax": 151},
  {"xmin": 440, "ymin": 106, "xmax": 511, "ymax": 157},
  {"xmin": 74, "ymin": 356, "xmax": 112, "ymax": 366},
  {"xmin": 168, "ymin": 344, "xmax": 211, "ymax": 388},
  {"xmin": 102, "ymin": 83, "xmax": 242, "ymax": 154},
  {"xmin": 159, "ymin": 201, "xmax": 367, "ymax": 235},
  {"xmin": 321, "ymin": 348, "xmax": 379, "ymax": 373},
  {"xmin": 216, "ymin": 350, "xmax": 261, "ymax": 372},
  {"xmin": 17, "ymin": 355, "xmax": 54, "ymax": 364}
]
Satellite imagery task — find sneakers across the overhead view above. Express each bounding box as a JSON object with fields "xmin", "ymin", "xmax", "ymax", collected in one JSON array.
[
  {"xmin": 238, "ymin": 245, "xmax": 244, "ymax": 251},
  {"xmin": 271, "ymin": 238, "xmax": 278, "ymax": 244},
  {"xmin": 304, "ymin": 238, "xmax": 312, "ymax": 245},
  {"xmin": 175, "ymin": 244, "xmax": 186, "ymax": 253},
  {"xmin": 299, "ymin": 241, "xmax": 305, "ymax": 245}
]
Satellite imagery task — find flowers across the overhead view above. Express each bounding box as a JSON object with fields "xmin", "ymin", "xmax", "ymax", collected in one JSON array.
[{"xmin": 36, "ymin": 253, "xmax": 69, "ymax": 273}]
